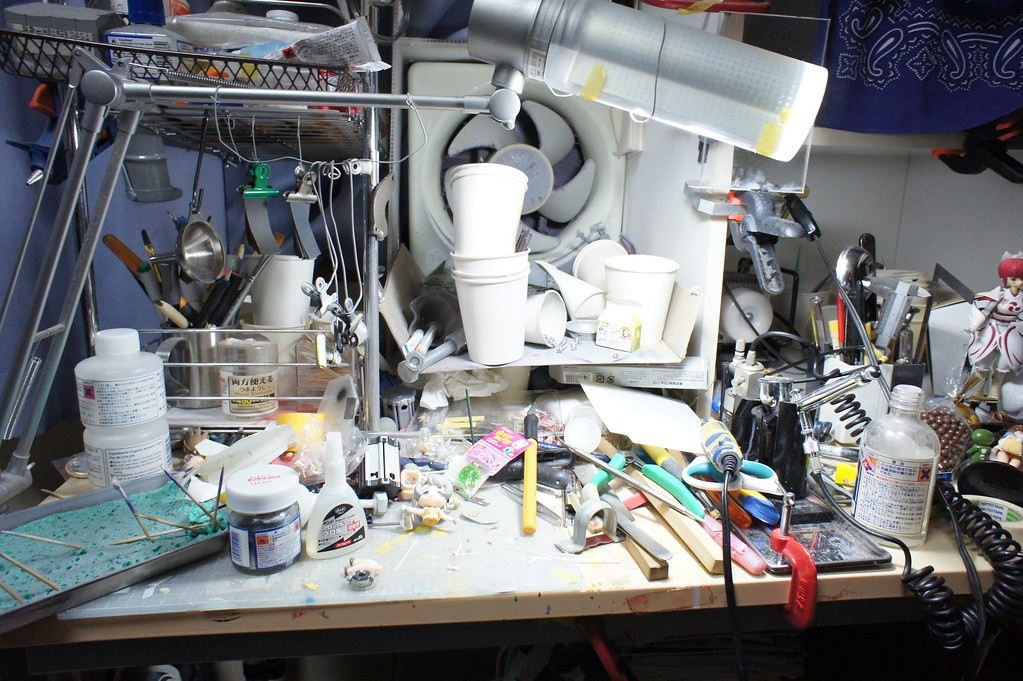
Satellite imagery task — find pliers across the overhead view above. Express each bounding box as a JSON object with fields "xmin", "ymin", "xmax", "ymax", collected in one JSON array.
[{"xmin": 577, "ymin": 443, "xmax": 705, "ymax": 523}]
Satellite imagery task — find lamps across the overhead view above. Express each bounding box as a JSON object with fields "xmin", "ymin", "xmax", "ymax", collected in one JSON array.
[{"xmin": 0, "ymin": 0, "xmax": 836, "ymax": 507}]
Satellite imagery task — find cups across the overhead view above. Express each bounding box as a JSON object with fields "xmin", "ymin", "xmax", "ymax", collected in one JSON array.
[
  {"xmin": 243, "ymin": 255, "xmax": 314, "ymax": 402},
  {"xmin": 571, "ymin": 239, "xmax": 629, "ymax": 293},
  {"xmin": 244, "ymin": 252, "xmax": 317, "ymax": 349},
  {"xmin": 604, "ymin": 253, "xmax": 680, "ymax": 342},
  {"xmin": 448, "ymin": 163, "xmax": 568, "ymax": 366},
  {"xmin": 156, "ymin": 325, "xmax": 235, "ymax": 408}
]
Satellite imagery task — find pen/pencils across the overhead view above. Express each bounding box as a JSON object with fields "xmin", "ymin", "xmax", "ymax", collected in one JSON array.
[
  {"xmin": 193, "ymin": 266, "xmax": 255, "ymax": 328},
  {"xmin": 154, "ymin": 299, "xmax": 194, "ymax": 328},
  {"xmin": 138, "ymin": 262, "xmax": 161, "ymax": 302},
  {"xmin": 177, "ymin": 296, "xmax": 199, "ymax": 325}
]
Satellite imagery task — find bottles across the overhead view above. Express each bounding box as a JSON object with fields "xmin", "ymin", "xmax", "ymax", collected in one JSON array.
[
  {"xmin": 850, "ymin": 384, "xmax": 941, "ymax": 550},
  {"xmin": 74, "ymin": 328, "xmax": 175, "ymax": 489},
  {"xmin": 226, "ymin": 464, "xmax": 302, "ymax": 576}
]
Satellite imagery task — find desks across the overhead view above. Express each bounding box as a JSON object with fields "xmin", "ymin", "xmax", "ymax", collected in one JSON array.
[{"xmin": 0, "ymin": 416, "xmax": 1021, "ymax": 680}]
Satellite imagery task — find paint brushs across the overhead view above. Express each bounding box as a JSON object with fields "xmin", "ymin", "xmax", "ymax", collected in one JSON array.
[{"xmin": 140, "ymin": 227, "xmax": 163, "ymax": 291}]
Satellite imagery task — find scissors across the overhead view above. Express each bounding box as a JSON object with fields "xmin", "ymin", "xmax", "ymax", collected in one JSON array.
[{"xmin": 682, "ymin": 452, "xmax": 779, "ymax": 492}]
